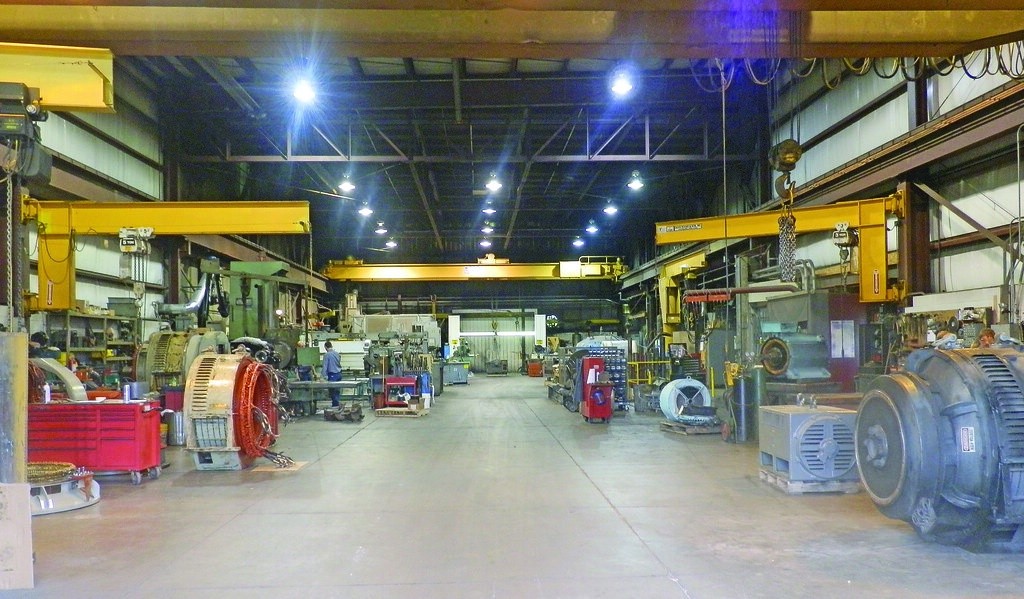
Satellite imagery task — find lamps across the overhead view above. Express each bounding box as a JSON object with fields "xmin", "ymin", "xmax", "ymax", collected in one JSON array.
[{"xmin": 338, "ymin": 168, "xmax": 644, "ymax": 249}]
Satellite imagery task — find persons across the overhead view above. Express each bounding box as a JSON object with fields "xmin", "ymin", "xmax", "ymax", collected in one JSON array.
[{"xmin": 320, "ymin": 341, "xmax": 342, "ymax": 407}]
